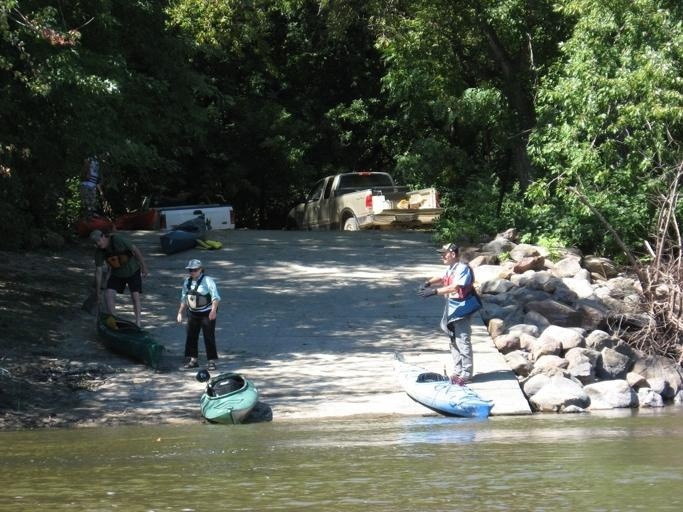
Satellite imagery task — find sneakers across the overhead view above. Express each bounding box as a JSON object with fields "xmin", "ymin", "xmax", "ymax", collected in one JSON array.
[
  {"xmin": 208, "ymin": 361, "xmax": 216, "ymax": 371},
  {"xmin": 179, "ymin": 361, "xmax": 200, "ymax": 372}
]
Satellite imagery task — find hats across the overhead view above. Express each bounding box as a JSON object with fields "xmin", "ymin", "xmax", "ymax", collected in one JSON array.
[
  {"xmin": 89, "ymin": 229, "xmax": 102, "ymax": 242},
  {"xmin": 436, "ymin": 243, "xmax": 459, "ymax": 253},
  {"xmin": 185, "ymin": 259, "xmax": 202, "ymax": 270}
]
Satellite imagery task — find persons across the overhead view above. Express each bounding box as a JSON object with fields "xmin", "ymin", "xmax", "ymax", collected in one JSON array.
[
  {"xmin": 90, "ymin": 230, "xmax": 149, "ymax": 329},
  {"xmin": 80, "ymin": 152, "xmax": 109, "ymax": 220},
  {"xmin": 416, "ymin": 243, "xmax": 481, "ymax": 384},
  {"xmin": 175, "ymin": 259, "xmax": 222, "ymax": 372}
]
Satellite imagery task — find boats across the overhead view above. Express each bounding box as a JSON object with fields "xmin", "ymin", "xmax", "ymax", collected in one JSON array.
[
  {"xmin": 117, "ymin": 209, "xmax": 156, "ymax": 229},
  {"xmin": 404, "ymin": 370, "xmax": 495, "ymax": 420},
  {"xmin": 160, "ymin": 213, "xmax": 206, "ymax": 255},
  {"xmin": 96, "ymin": 306, "xmax": 168, "ymax": 369},
  {"xmin": 199, "ymin": 371, "xmax": 259, "ymax": 424},
  {"xmin": 79, "ymin": 213, "xmax": 110, "ymax": 231}
]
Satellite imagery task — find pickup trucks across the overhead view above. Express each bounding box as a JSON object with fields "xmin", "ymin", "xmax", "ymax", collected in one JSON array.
[
  {"xmin": 128, "ymin": 191, "xmax": 237, "ymax": 230},
  {"xmin": 302, "ymin": 170, "xmax": 445, "ymax": 230}
]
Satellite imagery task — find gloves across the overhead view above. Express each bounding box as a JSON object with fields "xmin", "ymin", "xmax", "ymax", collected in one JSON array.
[{"xmin": 416, "ymin": 281, "xmax": 437, "ymax": 298}]
